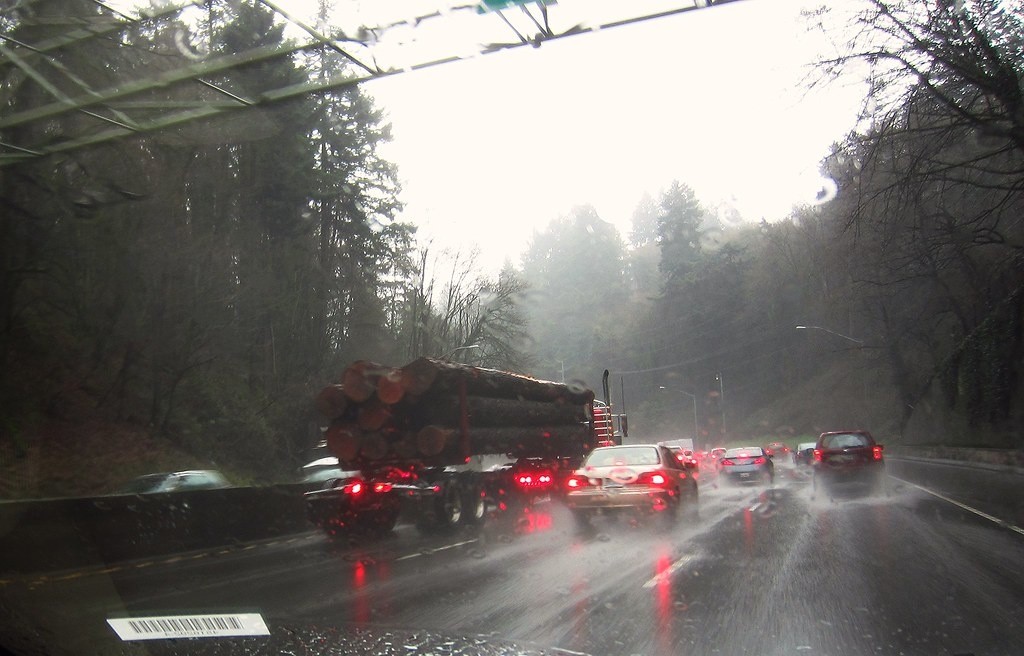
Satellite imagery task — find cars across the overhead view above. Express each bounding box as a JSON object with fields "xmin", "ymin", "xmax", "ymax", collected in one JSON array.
[
  {"xmin": 89, "ymin": 471, "xmax": 238, "ymax": 501},
  {"xmin": 814, "ymin": 429, "xmax": 885, "ymax": 489},
  {"xmin": 764, "ymin": 442, "xmax": 790, "ymax": 461},
  {"xmin": 662, "ymin": 442, "xmax": 729, "ymax": 485},
  {"xmin": 790, "ymin": 442, "xmax": 817, "ymax": 466},
  {"xmin": 722, "ymin": 447, "xmax": 776, "ymax": 484},
  {"xmin": 564, "ymin": 444, "xmax": 699, "ymax": 529}
]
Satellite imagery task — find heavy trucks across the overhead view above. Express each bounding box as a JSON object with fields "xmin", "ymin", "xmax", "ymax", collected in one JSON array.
[{"xmin": 298, "ymin": 366, "xmax": 629, "ymax": 544}]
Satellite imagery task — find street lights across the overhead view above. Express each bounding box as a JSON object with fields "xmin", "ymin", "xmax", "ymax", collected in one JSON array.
[
  {"xmin": 659, "ymin": 383, "xmax": 698, "ymax": 442},
  {"xmin": 795, "ymin": 323, "xmax": 870, "ymax": 430}
]
